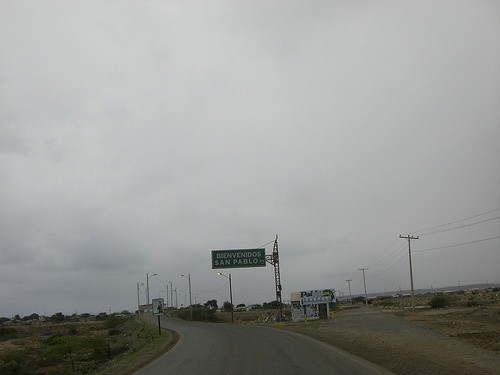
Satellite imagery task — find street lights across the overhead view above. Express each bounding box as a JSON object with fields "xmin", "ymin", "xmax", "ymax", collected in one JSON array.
[
  {"xmin": 217, "ymin": 271, "xmax": 234, "ymax": 324},
  {"xmin": 147, "ymin": 272, "xmax": 157, "ymax": 305},
  {"xmin": 180, "ymin": 273, "xmax": 192, "ymax": 321}
]
